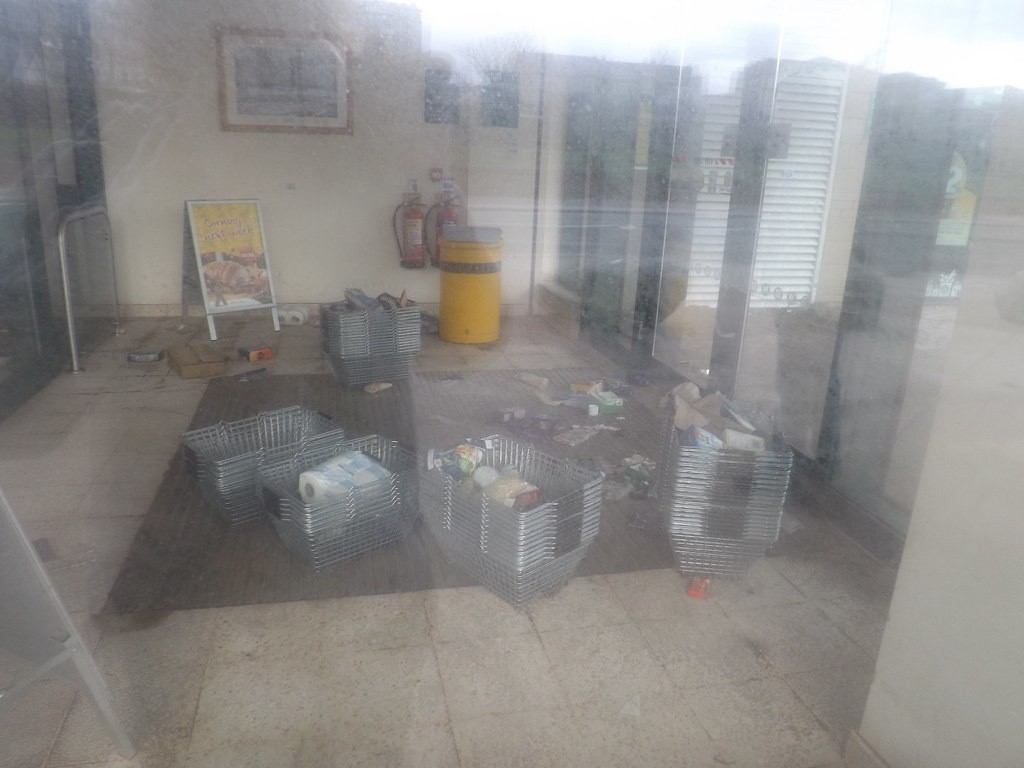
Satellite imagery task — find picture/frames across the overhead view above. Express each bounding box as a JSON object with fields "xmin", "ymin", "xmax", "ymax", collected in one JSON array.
[{"xmin": 214, "ymin": 24, "xmax": 355, "ymax": 136}]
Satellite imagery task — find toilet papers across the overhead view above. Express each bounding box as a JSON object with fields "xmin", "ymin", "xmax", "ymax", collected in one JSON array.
[{"xmin": 299, "ymin": 449, "xmax": 393, "ymax": 507}]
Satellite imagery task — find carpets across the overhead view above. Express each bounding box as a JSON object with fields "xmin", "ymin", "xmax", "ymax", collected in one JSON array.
[{"xmin": 93, "ymin": 363, "xmax": 850, "ymax": 621}]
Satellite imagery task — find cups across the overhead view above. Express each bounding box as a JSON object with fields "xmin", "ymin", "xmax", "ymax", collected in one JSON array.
[{"xmin": 588, "ymin": 405, "xmax": 599, "ymax": 417}]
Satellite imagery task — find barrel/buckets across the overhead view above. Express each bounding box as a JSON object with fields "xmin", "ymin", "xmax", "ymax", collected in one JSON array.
[{"xmin": 438, "ymin": 239, "xmax": 500, "ymax": 345}]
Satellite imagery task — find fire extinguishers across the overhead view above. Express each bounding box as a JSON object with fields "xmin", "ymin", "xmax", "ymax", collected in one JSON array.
[
  {"xmin": 424, "ymin": 196, "xmax": 461, "ymax": 262},
  {"xmin": 392, "ymin": 196, "xmax": 426, "ymax": 264}
]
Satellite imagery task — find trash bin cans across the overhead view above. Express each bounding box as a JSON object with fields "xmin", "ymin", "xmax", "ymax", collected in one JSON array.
[{"xmin": 436, "ymin": 238, "xmax": 501, "ymax": 343}]
